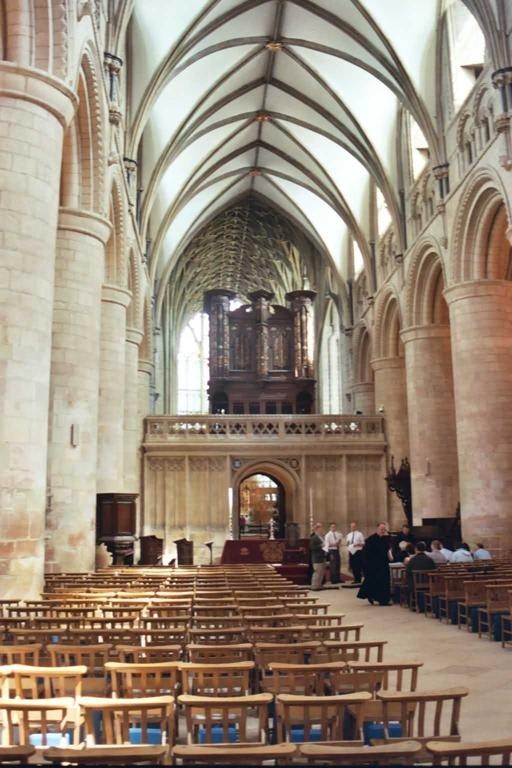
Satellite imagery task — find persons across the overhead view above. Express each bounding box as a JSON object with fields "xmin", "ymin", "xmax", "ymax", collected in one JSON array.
[
  {"xmin": 324, "ymin": 522, "xmax": 343, "ymax": 584},
  {"xmin": 239, "ymin": 516, "xmax": 247, "ymax": 535},
  {"xmin": 343, "ymin": 522, "xmax": 366, "ymax": 583},
  {"xmin": 392, "ymin": 525, "xmax": 492, "ymax": 611},
  {"xmin": 308, "ymin": 524, "xmax": 327, "ymax": 591},
  {"xmin": 364, "ymin": 521, "xmax": 393, "ymax": 606}
]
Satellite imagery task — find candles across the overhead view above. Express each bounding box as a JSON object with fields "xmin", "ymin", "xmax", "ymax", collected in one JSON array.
[
  {"xmin": 309, "ymin": 488, "xmax": 313, "ymax": 517},
  {"xmin": 227, "ymin": 487, "xmax": 234, "ymax": 519}
]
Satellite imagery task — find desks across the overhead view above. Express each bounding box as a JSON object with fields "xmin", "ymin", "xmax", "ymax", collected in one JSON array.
[{"xmin": 218, "ymin": 537, "xmax": 312, "ymax": 565}]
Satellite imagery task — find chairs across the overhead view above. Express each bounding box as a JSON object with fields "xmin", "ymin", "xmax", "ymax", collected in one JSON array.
[
  {"xmin": 78, "ymin": 695, "xmax": 175, "ymax": 750},
  {"xmin": 389, "ymin": 559, "xmax": 511, "ymax": 649},
  {"xmin": 0, "ymin": 744, "xmax": 37, "ymax": 767},
  {"xmin": 2, "ymin": 697, "xmax": 86, "ymax": 763},
  {"xmin": 176, "ymin": 692, "xmax": 273, "ymax": 745},
  {"xmin": 426, "ymin": 739, "xmax": 511, "ymax": 767},
  {"xmin": 173, "ymin": 742, "xmax": 299, "ymax": 767},
  {"xmin": 276, "ymin": 691, "xmax": 372, "ymax": 746},
  {"xmin": 368, "ymin": 685, "xmax": 470, "ymax": 746},
  {"xmin": 1, "ymin": 559, "xmax": 424, "ymax": 736},
  {"xmin": 299, "ymin": 739, "xmax": 424, "ymax": 768},
  {"xmin": 42, "ymin": 743, "xmax": 170, "ymax": 768}
]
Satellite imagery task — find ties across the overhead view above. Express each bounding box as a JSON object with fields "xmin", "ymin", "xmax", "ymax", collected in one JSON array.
[
  {"xmin": 351, "ymin": 532, "xmax": 355, "ymax": 545},
  {"xmin": 333, "ymin": 534, "xmax": 337, "ymax": 542}
]
